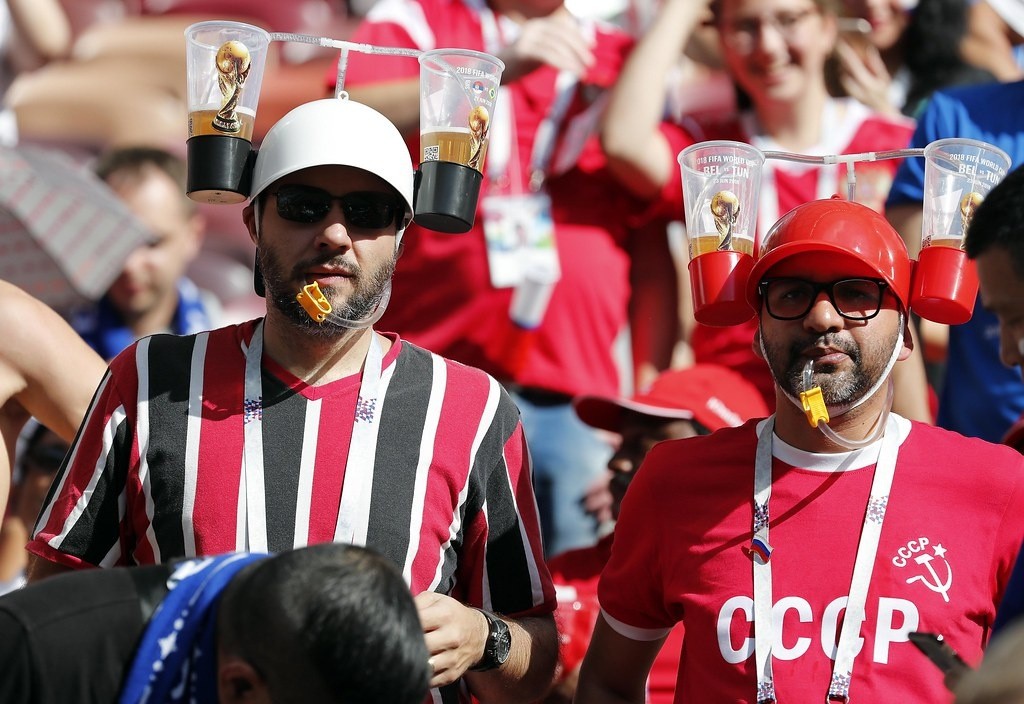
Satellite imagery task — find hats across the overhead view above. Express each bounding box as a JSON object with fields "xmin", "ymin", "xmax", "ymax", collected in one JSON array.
[{"xmin": 574, "ymin": 364, "xmax": 770, "ymax": 433}]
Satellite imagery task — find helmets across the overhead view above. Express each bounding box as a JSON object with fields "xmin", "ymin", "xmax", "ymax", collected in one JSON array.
[
  {"xmin": 246, "ymin": 98, "xmax": 415, "ymax": 227},
  {"xmin": 754, "ymin": 193, "xmax": 911, "ymax": 320}
]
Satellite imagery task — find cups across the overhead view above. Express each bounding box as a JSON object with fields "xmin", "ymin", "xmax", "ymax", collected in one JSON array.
[
  {"xmin": 676, "ymin": 139, "xmax": 767, "ymax": 327},
  {"xmin": 414, "ymin": 47, "xmax": 506, "ymax": 234},
  {"xmin": 909, "ymin": 137, "xmax": 1012, "ymax": 325},
  {"xmin": 185, "ymin": 19, "xmax": 272, "ymax": 204}
]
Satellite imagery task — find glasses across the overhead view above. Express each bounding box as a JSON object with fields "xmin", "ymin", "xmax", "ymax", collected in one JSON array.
[
  {"xmin": 269, "ymin": 184, "xmax": 404, "ymax": 230},
  {"xmin": 757, "ymin": 277, "xmax": 888, "ymax": 320},
  {"xmin": 714, "ymin": 3, "xmax": 818, "ymax": 59}
]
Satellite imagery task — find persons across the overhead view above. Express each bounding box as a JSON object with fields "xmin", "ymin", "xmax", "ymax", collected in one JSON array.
[
  {"xmin": 1, "ymin": 91, "xmax": 559, "ymax": 701},
  {"xmin": 0, "ymin": 0, "xmax": 1024, "ymax": 603},
  {"xmin": 1, "ymin": 278, "xmax": 111, "ymax": 596},
  {"xmin": 569, "ymin": 194, "xmax": 1023, "ymax": 702}
]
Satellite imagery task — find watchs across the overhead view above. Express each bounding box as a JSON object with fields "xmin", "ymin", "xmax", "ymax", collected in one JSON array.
[{"xmin": 470, "ymin": 605, "xmax": 513, "ymax": 675}]
